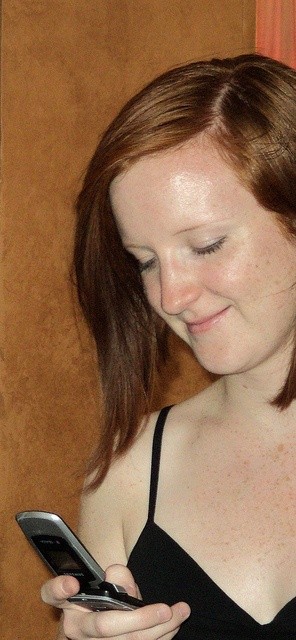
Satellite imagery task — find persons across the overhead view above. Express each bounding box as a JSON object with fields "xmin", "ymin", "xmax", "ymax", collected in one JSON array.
[{"xmin": 40, "ymin": 54, "xmax": 296, "ymax": 639}]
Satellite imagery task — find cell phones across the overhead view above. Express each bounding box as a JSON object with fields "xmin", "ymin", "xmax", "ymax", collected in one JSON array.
[{"xmin": 16, "ymin": 510, "xmax": 147, "ymax": 612}]
